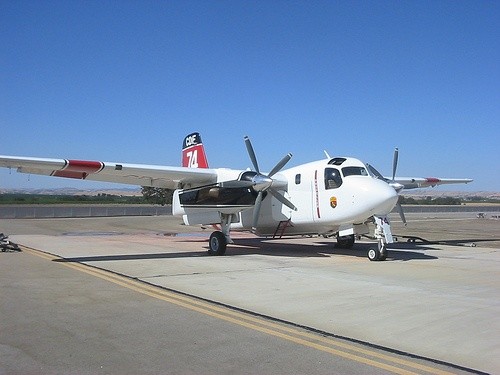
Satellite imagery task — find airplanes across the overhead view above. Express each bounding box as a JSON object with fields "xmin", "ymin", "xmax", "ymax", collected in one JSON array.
[{"xmin": 0, "ymin": 132, "xmax": 474, "ymax": 261}]
